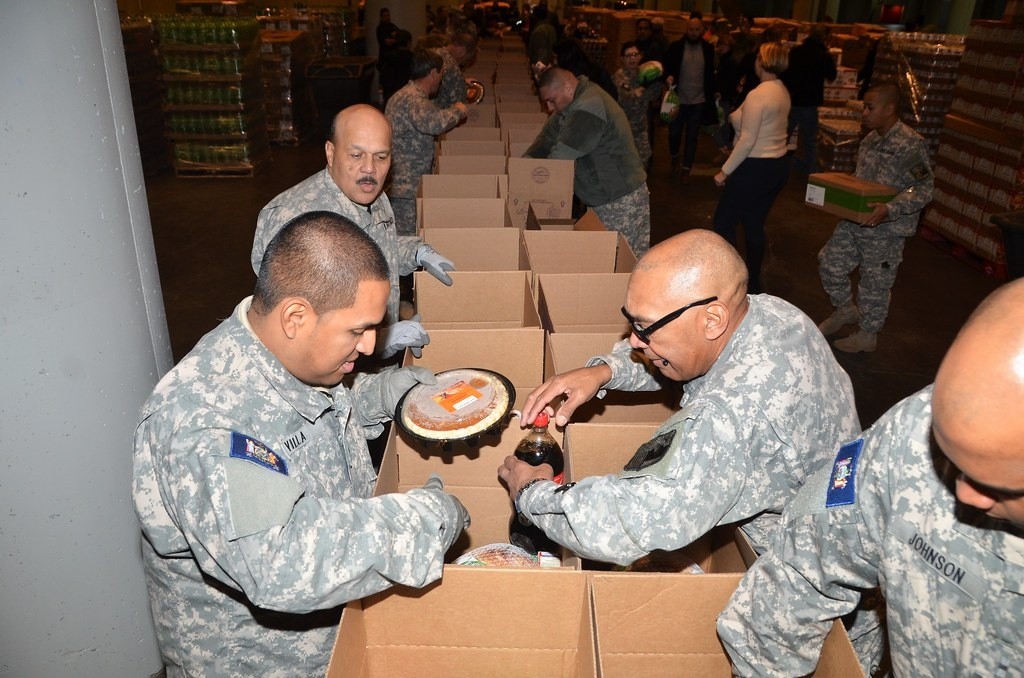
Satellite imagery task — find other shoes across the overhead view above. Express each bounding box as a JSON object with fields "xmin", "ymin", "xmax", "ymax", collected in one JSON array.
[
  {"xmin": 672, "ymin": 155, "xmax": 681, "ymax": 173},
  {"xmin": 719, "ymin": 146, "xmax": 730, "ymax": 157},
  {"xmin": 679, "ymin": 169, "xmax": 690, "ymax": 186}
]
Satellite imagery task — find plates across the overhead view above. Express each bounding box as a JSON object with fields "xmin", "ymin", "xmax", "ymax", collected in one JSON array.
[{"xmin": 393, "ymin": 368, "xmax": 516, "ymax": 441}]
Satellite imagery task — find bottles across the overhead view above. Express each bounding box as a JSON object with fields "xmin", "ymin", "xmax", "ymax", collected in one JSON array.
[
  {"xmin": 509, "ymin": 411, "xmax": 565, "ymax": 556},
  {"xmin": 157, "ymin": 14, "xmax": 259, "ymax": 165},
  {"xmin": 265, "ymin": 5, "xmax": 355, "ymax": 59}
]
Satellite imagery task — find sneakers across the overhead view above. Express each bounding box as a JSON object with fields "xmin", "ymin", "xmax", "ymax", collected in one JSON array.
[
  {"xmin": 834, "ymin": 329, "xmax": 877, "ymax": 353},
  {"xmin": 818, "ymin": 306, "xmax": 860, "ymax": 335}
]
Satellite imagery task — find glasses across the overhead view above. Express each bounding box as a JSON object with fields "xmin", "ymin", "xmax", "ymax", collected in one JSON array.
[
  {"xmin": 623, "ymin": 51, "xmax": 642, "ymax": 58},
  {"xmin": 638, "ymin": 26, "xmax": 651, "ymax": 31},
  {"xmin": 929, "ymin": 422, "xmax": 1024, "ymax": 502},
  {"xmin": 621, "ymin": 296, "xmax": 718, "ymax": 345}
]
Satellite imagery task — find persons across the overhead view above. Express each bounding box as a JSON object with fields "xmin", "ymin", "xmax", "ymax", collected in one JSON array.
[
  {"xmin": 377, "ymin": 7, "xmax": 880, "ymax": 294},
  {"xmin": 252, "ymin": 104, "xmax": 456, "ymax": 374},
  {"xmin": 818, "ymin": 81, "xmax": 934, "ymax": 353},
  {"xmin": 715, "ymin": 277, "xmax": 1024, "ymax": 678},
  {"xmin": 132, "ymin": 211, "xmax": 471, "ymax": 678},
  {"xmin": 497, "ymin": 229, "xmax": 888, "ymax": 678}
]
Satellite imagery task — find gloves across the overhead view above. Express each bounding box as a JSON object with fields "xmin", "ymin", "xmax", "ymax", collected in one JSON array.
[
  {"xmin": 640, "ymin": 73, "xmax": 658, "ymax": 89},
  {"xmin": 369, "ymin": 313, "xmax": 430, "ymax": 360},
  {"xmin": 388, "ymin": 365, "xmax": 436, "ymax": 423},
  {"xmin": 422, "ymin": 473, "xmax": 472, "ymax": 544},
  {"xmin": 413, "ymin": 243, "xmax": 456, "ymax": 287}
]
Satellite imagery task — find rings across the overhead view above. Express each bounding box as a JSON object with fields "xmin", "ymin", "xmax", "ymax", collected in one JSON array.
[{"xmin": 872, "ymin": 224, "xmax": 874, "ymax": 226}]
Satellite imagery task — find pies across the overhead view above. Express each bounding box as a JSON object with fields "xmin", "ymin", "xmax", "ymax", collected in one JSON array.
[{"xmin": 403, "ymin": 371, "xmax": 510, "ymax": 439}]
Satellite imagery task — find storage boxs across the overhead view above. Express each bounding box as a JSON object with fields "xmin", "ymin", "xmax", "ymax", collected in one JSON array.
[
  {"xmin": 919, "ymin": 19, "xmax": 1024, "ymax": 263},
  {"xmin": 324, "ymin": 6, "xmax": 902, "ymax": 678}
]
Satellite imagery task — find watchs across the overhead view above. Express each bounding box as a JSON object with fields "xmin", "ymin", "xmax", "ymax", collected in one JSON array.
[{"xmin": 514, "ymin": 478, "xmax": 548, "ymax": 523}]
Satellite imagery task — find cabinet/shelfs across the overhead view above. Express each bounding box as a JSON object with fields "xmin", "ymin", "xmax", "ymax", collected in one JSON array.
[
  {"xmin": 262, "ymin": 27, "xmax": 324, "ymax": 149},
  {"xmin": 157, "ymin": 37, "xmax": 273, "ymax": 177}
]
